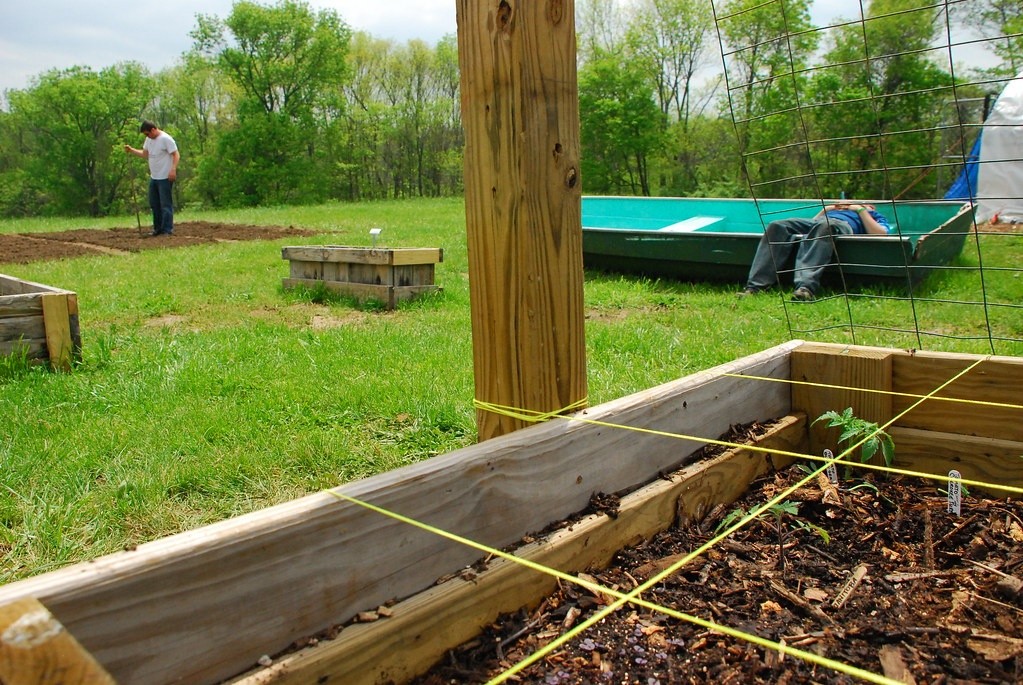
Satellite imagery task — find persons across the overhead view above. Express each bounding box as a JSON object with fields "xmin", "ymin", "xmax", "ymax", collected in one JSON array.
[
  {"xmin": 746, "ymin": 204, "xmax": 891, "ymax": 301},
  {"xmin": 125, "ymin": 121, "xmax": 180, "ymax": 236}
]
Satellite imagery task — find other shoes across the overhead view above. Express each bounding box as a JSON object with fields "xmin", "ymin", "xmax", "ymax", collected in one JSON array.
[
  {"xmin": 792, "ymin": 286, "xmax": 813, "ymax": 302},
  {"xmin": 737, "ymin": 287, "xmax": 757, "ymax": 296},
  {"xmin": 149, "ymin": 232, "xmax": 159, "ymax": 236},
  {"xmin": 158, "ymin": 233, "xmax": 171, "ymax": 237}
]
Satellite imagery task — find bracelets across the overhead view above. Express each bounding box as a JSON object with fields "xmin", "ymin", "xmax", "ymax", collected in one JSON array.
[{"xmin": 856, "ymin": 207, "xmax": 866, "ymax": 213}]
